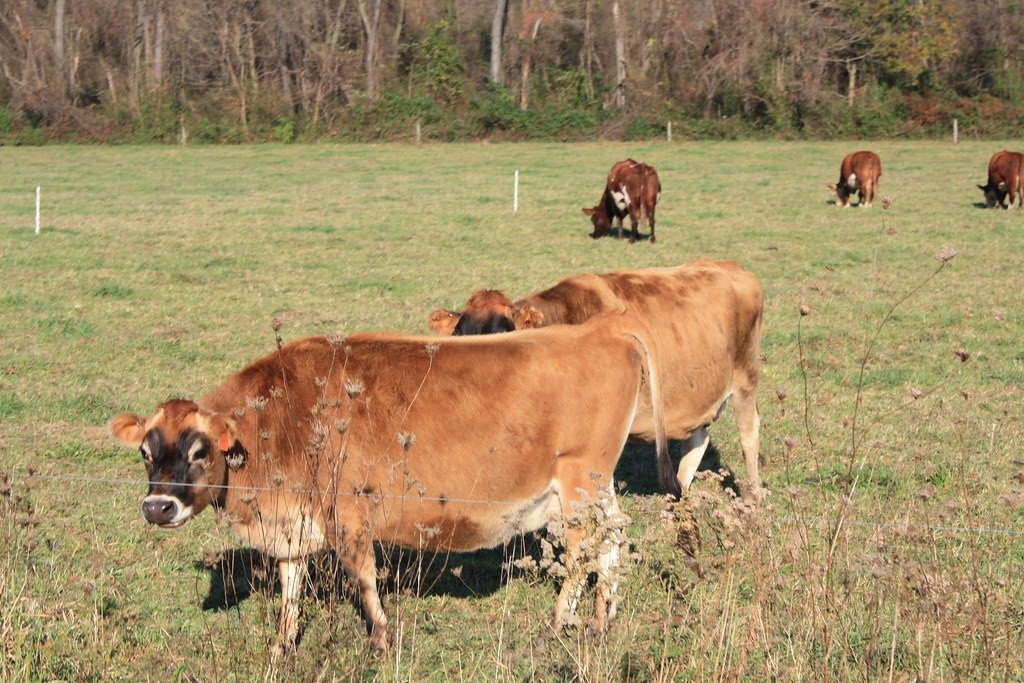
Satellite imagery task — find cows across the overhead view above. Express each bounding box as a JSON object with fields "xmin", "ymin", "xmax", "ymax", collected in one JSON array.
[
  {"xmin": 975, "ymin": 149, "xmax": 1024, "ymax": 210},
  {"xmin": 581, "ymin": 159, "xmax": 662, "ymax": 244},
  {"xmin": 110, "ymin": 310, "xmax": 682, "ymax": 682},
  {"xmin": 825, "ymin": 150, "xmax": 882, "ymax": 209},
  {"xmin": 428, "ymin": 258, "xmax": 765, "ymax": 510}
]
